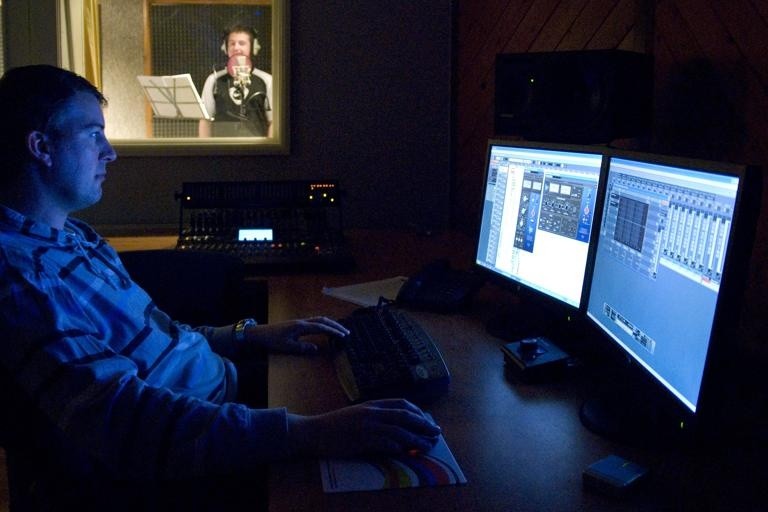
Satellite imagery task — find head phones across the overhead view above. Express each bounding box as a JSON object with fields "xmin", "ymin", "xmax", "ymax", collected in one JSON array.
[{"xmin": 220, "ymin": 24, "xmax": 261, "ymax": 57}]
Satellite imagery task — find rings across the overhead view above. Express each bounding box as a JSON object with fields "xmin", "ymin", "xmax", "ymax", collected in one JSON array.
[{"xmin": 310, "ymin": 316, "xmax": 316, "ymax": 323}]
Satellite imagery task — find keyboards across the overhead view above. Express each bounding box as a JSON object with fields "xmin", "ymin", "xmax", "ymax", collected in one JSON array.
[{"xmin": 328, "ymin": 305, "xmax": 451, "ymax": 401}]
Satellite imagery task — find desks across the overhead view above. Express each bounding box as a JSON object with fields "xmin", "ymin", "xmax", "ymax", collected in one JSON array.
[{"xmin": 80, "ymin": 227, "xmax": 768, "ymax": 511}]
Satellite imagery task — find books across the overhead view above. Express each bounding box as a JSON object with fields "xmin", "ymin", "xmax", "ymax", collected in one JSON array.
[{"xmin": 135, "ymin": 73, "xmax": 210, "ymax": 121}]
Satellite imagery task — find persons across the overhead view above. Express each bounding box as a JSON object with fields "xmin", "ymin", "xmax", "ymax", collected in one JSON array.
[
  {"xmin": 0, "ymin": 64, "xmax": 443, "ymax": 511},
  {"xmin": 196, "ymin": 23, "xmax": 275, "ymax": 139}
]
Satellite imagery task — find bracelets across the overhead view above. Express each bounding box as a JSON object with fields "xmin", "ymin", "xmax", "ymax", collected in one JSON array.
[{"xmin": 235, "ymin": 316, "xmax": 258, "ymax": 344}]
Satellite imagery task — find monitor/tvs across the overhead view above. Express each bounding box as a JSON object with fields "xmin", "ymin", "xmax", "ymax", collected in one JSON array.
[
  {"xmin": 579, "ymin": 155, "xmax": 762, "ymax": 444},
  {"xmin": 470, "ymin": 134, "xmax": 610, "ymax": 342}
]
